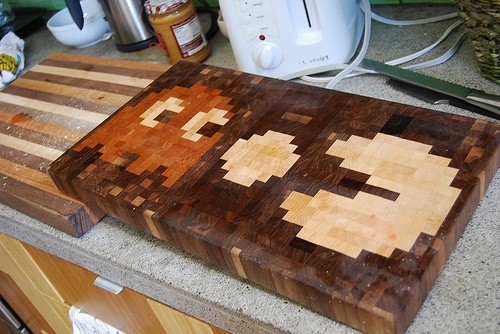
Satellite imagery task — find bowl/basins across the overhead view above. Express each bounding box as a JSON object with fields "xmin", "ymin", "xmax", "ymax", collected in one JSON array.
[{"xmin": 45, "ymin": 0, "xmax": 108, "ymax": 49}]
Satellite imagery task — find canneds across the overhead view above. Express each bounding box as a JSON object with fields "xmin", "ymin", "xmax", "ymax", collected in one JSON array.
[{"xmin": 144, "ymin": 0, "xmax": 211, "ymax": 63}]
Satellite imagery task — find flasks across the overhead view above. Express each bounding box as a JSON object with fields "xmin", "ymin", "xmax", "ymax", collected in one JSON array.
[{"xmin": 99, "ymin": 1, "xmax": 155, "ymax": 53}]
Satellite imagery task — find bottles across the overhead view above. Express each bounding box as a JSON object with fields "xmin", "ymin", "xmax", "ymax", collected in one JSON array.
[{"xmin": 1, "ymin": 1, "xmax": 13, "ymax": 26}]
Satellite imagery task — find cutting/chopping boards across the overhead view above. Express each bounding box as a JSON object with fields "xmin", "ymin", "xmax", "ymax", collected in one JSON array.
[
  {"xmin": 48, "ymin": 59, "xmax": 499, "ymax": 333},
  {"xmin": 2, "ymin": 54, "xmax": 172, "ymax": 237}
]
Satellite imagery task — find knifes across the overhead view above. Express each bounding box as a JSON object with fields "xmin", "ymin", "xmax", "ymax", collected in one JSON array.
[{"xmin": 362, "ymin": 53, "xmax": 498, "ymax": 122}]
